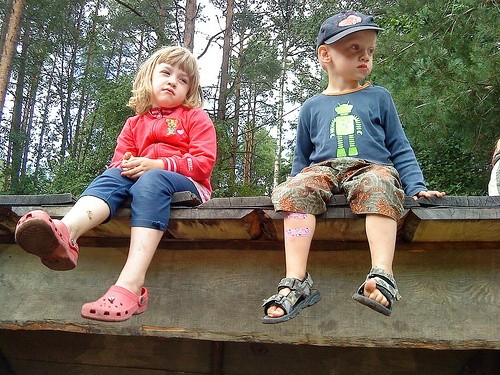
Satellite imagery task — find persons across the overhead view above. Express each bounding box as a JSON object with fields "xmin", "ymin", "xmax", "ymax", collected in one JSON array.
[
  {"xmin": 15, "ymin": 46, "xmax": 217, "ymax": 322},
  {"xmin": 488, "ymin": 139, "xmax": 500, "ymax": 196},
  {"xmin": 261, "ymin": 10, "xmax": 446, "ymax": 325}
]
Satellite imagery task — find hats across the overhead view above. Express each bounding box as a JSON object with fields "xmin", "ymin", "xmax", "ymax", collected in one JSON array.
[{"xmin": 316, "ymin": 10, "xmax": 384, "ymax": 54}]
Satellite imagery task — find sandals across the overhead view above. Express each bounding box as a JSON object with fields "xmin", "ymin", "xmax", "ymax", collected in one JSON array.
[
  {"xmin": 15, "ymin": 210, "xmax": 79, "ymax": 271},
  {"xmin": 352, "ymin": 267, "xmax": 402, "ymax": 316},
  {"xmin": 262, "ymin": 271, "xmax": 322, "ymax": 324},
  {"xmin": 81, "ymin": 284, "xmax": 148, "ymax": 321}
]
